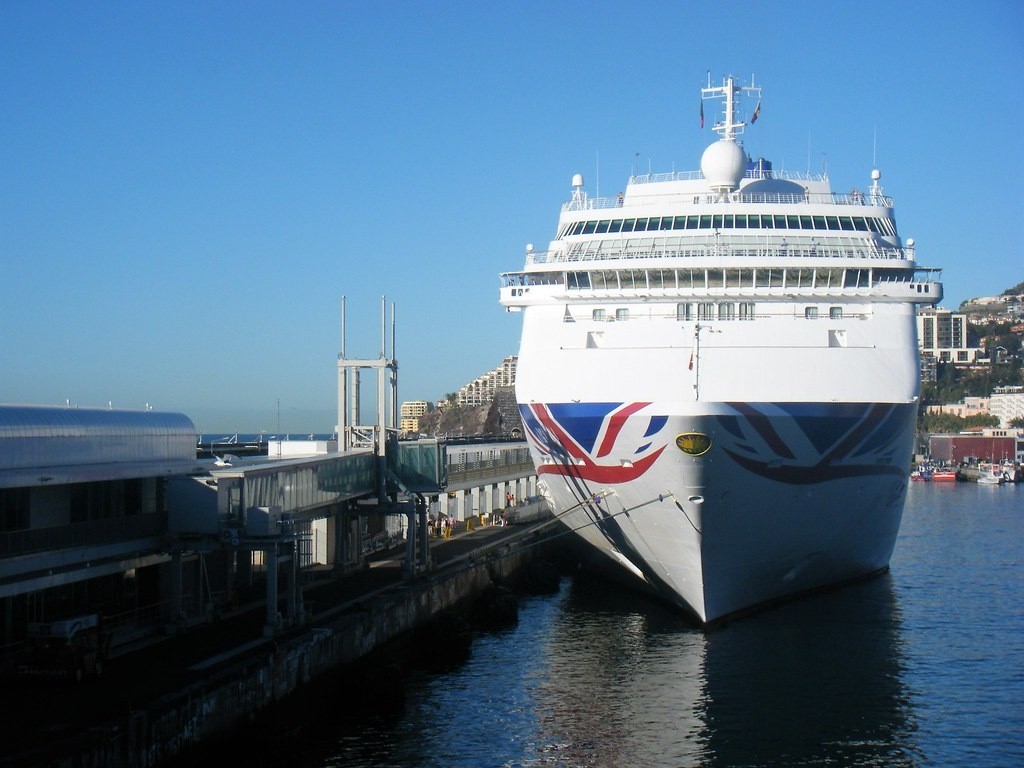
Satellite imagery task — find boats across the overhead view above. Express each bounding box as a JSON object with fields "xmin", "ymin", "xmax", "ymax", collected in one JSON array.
[{"xmin": 911, "ymin": 464, "xmax": 957, "ymax": 482}]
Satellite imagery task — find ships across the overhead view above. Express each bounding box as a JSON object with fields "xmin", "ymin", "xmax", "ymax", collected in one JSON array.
[{"xmin": 496, "ymin": 70, "xmax": 944, "ymax": 628}]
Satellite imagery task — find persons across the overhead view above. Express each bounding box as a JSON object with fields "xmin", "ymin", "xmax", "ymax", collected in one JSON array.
[
  {"xmin": 619, "ymin": 192, "xmax": 624, "ymax": 207},
  {"xmin": 861, "ymin": 193, "xmax": 865, "ymax": 205},
  {"xmin": 427, "ymin": 514, "xmax": 456, "ymax": 535},
  {"xmin": 506, "ymin": 492, "xmax": 514, "ymax": 507},
  {"xmin": 781, "ymin": 238, "xmax": 787, "ymax": 247},
  {"xmin": 501, "ymin": 511, "xmax": 506, "ymax": 526},
  {"xmin": 810, "ymin": 237, "xmax": 820, "ymax": 256},
  {"xmin": 519, "ymin": 274, "xmax": 524, "ymax": 286},
  {"xmin": 852, "ymin": 189, "xmax": 858, "ymax": 204},
  {"xmin": 804, "ymin": 186, "xmax": 809, "ymax": 200}
]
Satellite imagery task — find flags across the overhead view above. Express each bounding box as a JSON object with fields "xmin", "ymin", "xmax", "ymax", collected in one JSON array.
[
  {"xmin": 700, "ymin": 99, "xmax": 704, "ymax": 128},
  {"xmin": 751, "ymin": 101, "xmax": 761, "ymax": 125}
]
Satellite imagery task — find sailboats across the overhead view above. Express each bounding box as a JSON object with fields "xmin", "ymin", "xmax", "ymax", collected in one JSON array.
[{"xmin": 976, "ymin": 442, "xmax": 1002, "ymax": 484}]
